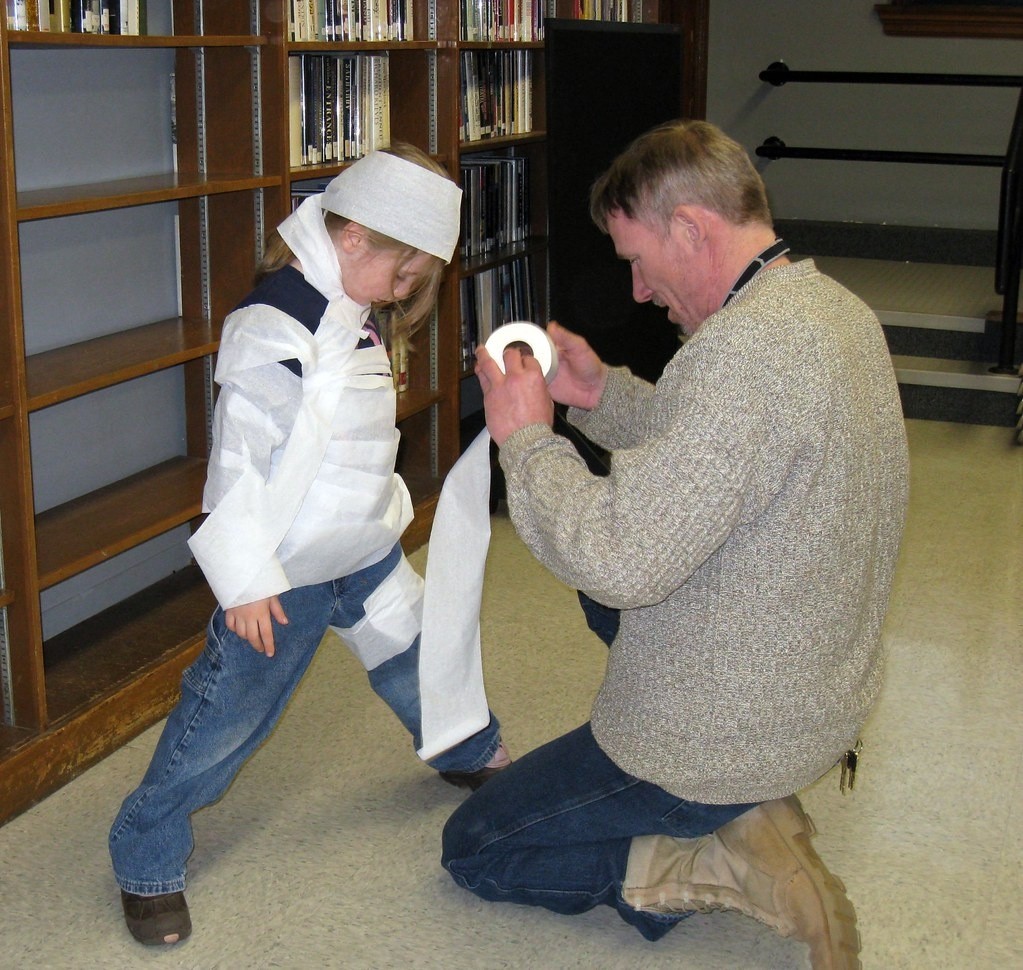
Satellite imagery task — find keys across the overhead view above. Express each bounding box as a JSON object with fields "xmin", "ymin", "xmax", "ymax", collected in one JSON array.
[{"xmin": 839, "ymin": 750, "xmax": 858, "ymax": 795}]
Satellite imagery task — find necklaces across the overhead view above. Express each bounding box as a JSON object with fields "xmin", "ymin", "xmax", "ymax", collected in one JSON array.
[{"xmin": 720, "ymin": 237, "xmax": 790, "ymax": 309}]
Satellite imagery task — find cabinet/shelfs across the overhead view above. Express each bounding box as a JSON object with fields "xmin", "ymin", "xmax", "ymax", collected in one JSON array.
[
  {"xmin": 753, "ymin": 61, "xmax": 1022, "ymax": 428},
  {"xmin": 0, "ymin": 0, "xmax": 706, "ymax": 829}
]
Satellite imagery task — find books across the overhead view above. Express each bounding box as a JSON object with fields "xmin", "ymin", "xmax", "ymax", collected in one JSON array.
[
  {"xmin": 291, "ymin": 183, "xmax": 329, "ymax": 215},
  {"xmin": 460, "ymin": 148, "xmax": 528, "ymax": 258},
  {"xmin": 459, "ymin": 50, "xmax": 533, "ymax": 142},
  {"xmin": 571, "ymin": 0, "xmax": 642, "ymax": 23},
  {"xmin": 288, "ymin": 0, "xmax": 413, "ymax": 42},
  {"xmin": 289, "ymin": 51, "xmax": 392, "ymax": 168},
  {"xmin": 379, "ymin": 311, "xmax": 411, "ymax": 394},
  {"xmin": 459, "ymin": 0, "xmax": 557, "ymax": 41},
  {"xmin": 7, "ymin": 0, "xmax": 149, "ymax": 35},
  {"xmin": 461, "ymin": 258, "xmax": 529, "ymax": 372}
]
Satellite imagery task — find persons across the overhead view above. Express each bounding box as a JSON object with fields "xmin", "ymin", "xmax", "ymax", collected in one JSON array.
[
  {"xmin": 107, "ymin": 144, "xmax": 511, "ymax": 946},
  {"xmin": 441, "ymin": 120, "xmax": 914, "ymax": 970}
]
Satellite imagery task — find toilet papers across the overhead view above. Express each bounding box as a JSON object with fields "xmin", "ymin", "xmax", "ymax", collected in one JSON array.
[{"xmin": 486, "ymin": 321, "xmax": 560, "ymax": 388}]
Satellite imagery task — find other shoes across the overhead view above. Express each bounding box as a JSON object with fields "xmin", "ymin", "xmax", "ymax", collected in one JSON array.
[
  {"xmin": 440, "ymin": 764, "xmax": 510, "ymax": 792},
  {"xmin": 121, "ymin": 890, "xmax": 191, "ymax": 947}
]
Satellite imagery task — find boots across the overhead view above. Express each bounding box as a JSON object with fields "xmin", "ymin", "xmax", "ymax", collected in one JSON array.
[{"xmin": 619, "ymin": 796, "xmax": 863, "ymax": 970}]
